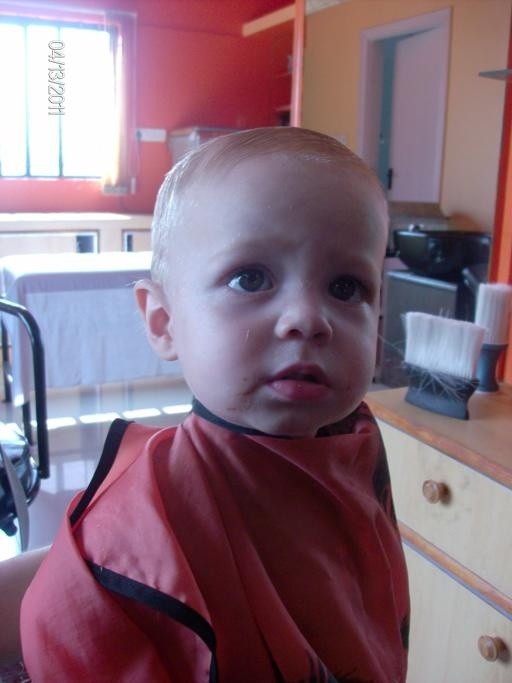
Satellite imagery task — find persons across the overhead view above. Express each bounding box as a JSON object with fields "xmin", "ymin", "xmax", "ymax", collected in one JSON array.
[{"xmin": 20, "ymin": 124, "xmax": 411, "ymax": 682}]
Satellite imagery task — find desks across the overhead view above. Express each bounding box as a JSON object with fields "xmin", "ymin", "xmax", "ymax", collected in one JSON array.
[{"xmin": 1, "ymin": 249, "xmax": 181, "ymax": 444}]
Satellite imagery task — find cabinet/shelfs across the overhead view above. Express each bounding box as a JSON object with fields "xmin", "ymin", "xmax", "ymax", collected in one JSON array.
[
  {"xmin": 363, "ymin": 379, "xmax": 512, "ymax": 682},
  {"xmin": 274, "ymin": 70, "xmax": 292, "ymax": 112}
]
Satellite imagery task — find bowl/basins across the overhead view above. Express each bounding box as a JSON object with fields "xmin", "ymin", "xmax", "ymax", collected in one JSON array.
[{"xmin": 391, "ymin": 229, "xmax": 491, "ymax": 280}]
